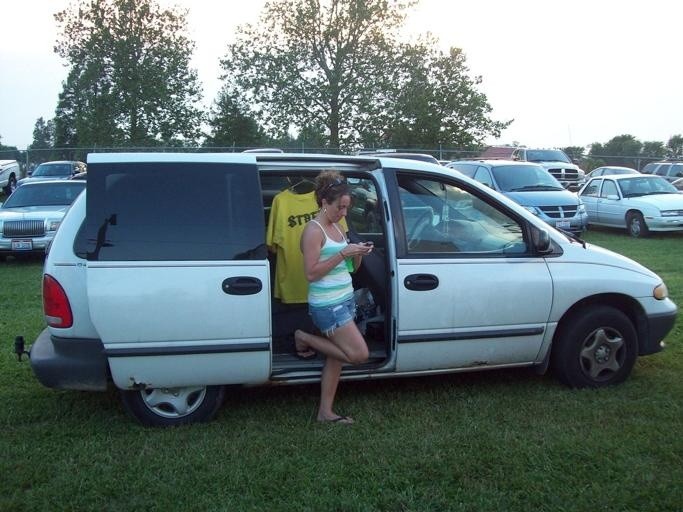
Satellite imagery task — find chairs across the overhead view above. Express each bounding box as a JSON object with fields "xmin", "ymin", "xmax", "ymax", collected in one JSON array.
[{"xmin": 343, "ymin": 215, "xmax": 392, "ymax": 315}]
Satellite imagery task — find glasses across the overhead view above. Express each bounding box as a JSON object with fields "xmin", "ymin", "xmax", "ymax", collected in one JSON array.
[{"xmin": 322, "ymin": 179, "xmax": 347, "ymax": 197}]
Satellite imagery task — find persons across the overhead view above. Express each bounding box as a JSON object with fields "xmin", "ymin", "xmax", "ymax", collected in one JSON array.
[{"xmin": 290, "ymin": 170, "xmax": 374, "ymax": 425}]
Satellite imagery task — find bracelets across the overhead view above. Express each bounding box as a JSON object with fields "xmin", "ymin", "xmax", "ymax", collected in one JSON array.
[{"xmin": 339, "ymin": 250, "xmax": 345, "ymax": 260}]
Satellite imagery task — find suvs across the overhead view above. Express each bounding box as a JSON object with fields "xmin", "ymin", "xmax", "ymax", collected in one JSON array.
[
  {"xmin": 15, "ymin": 145, "xmax": 680, "ymax": 420},
  {"xmin": 510, "ymin": 144, "xmax": 586, "ymax": 190}
]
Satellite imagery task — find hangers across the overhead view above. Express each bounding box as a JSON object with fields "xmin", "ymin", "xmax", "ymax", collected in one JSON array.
[{"xmin": 280, "ymin": 169, "xmax": 322, "ymax": 194}]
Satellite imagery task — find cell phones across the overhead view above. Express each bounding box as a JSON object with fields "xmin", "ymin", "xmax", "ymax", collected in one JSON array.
[{"xmin": 363, "ymin": 241, "xmax": 374, "ymax": 247}]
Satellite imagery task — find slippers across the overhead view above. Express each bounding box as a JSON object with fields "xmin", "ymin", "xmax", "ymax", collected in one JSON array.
[
  {"xmin": 286, "ymin": 339, "xmax": 317, "ymax": 360},
  {"xmin": 332, "ymin": 416, "xmax": 351, "ymax": 424}
]
